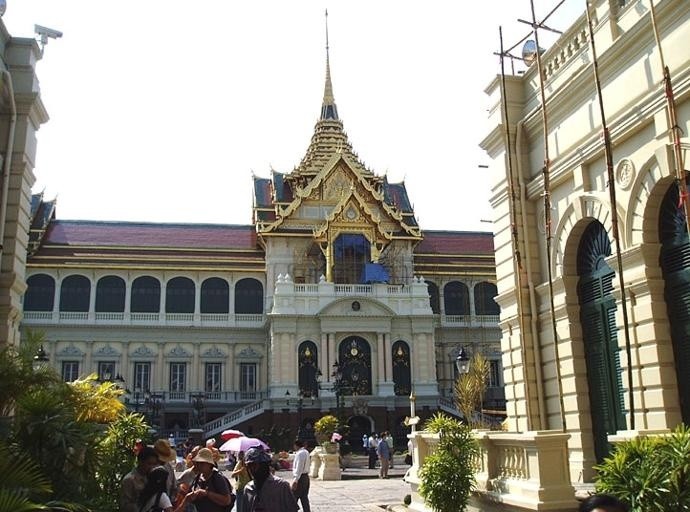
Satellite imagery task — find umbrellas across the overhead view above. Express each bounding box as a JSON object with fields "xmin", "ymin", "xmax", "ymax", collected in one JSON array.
[{"xmin": 221, "ymin": 428, "xmax": 244, "ymax": 442}]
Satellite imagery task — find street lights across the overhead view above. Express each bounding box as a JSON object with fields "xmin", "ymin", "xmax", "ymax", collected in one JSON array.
[
  {"xmin": 455, "ymin": 345, "xmax": 474, "ymax": 426},
  {"xmin": 103, "ymin": 368, "xmax": 133, "ymax": 405},
  {"xmin": 316, "ymin": 360, "xmax": 363, "ymax": 419},
  {"xmin": 127, "ymin": 382, "xmax": 152, "ymax": 412},
  {"xmin": 32, "ymin": 341, "xmax": 51, "ymax": 374}
]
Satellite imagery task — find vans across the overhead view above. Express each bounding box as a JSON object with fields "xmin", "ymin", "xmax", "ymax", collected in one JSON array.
[{"xmin": 141, "ymin": 429, "xmax": 161, "ymax": 446}]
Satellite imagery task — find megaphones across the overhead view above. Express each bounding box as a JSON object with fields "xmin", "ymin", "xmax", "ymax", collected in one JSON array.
[{"xmin": 522, "ymin": 40, "xmax": 546, "ymax": 67}]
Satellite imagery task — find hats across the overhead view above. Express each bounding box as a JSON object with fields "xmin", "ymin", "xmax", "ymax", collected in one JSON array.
[
  {"xmin": 191, "ymin": 447, "xmax": 216, "ymax": 466},
  {"xmin": 150, "ymin": 437, "xmax": 177, "ymax": 463},
  {"xmin": 242, "ymin": 447, "xmax": 273, "ymax": 465}
]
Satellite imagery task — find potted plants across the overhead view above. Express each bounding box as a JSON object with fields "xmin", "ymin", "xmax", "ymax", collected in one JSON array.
[{"xmin": 314, "ymin": 415, "xmax": 341, "ymax": 455}]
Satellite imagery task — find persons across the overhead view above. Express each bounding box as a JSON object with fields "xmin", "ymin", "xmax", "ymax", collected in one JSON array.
[
  {"xmin": 578, "ymin": 495, "xmax": 627, "ymax": 512},
  {"xmin": 118, "ymin": 434, "xmax": 310, "ymax": 512},
  {"xmin": 363, "ymin": 430, "xmax": 394, "ymax": 478},
  {"xmin": 334, "ymin": 439, "xmax": 345, "ymax": 471}
]
